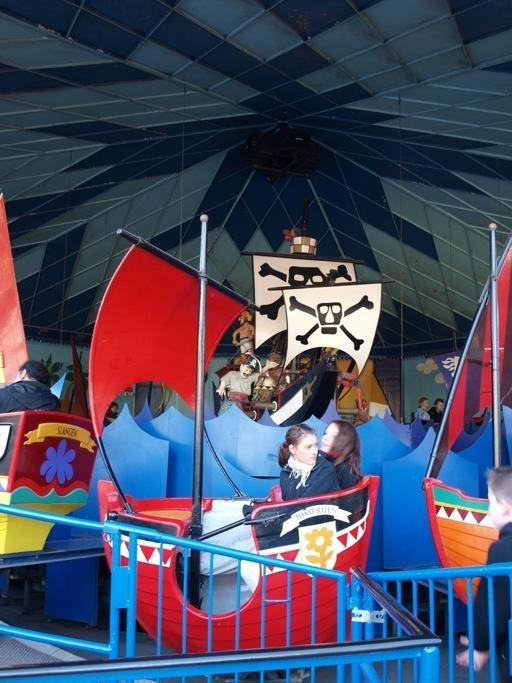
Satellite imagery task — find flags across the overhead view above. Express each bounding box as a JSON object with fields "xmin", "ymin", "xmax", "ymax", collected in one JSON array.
[{"xmin": 426, "ymin": 234, "xmax": 512, "ymax": 478}]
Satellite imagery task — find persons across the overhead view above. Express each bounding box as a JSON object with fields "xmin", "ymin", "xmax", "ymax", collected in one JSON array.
[
  {"xmin": 0, "ymin": 359, "xmax": 61, "ymax": 413},
  {"xmin": 250, "ymin": 351, "xmax": 310, "ymax": 415},
  {"xmin": 451, "ymin": 461, "xmax": 512, "ymax": 682},
  {"xmin": 215, "ymin": 353, "xmax": 281, "ymax": 418},
  {"xmin": 428, "ymin": 397, "xmax": 446, "ymax": 430},
  {"xmin": 232, "ymin": 308, "xmax": 254, "ymax": 363},
  {"xmin": 242, "ymin": 421, "xmax": 352, "ymax": 550},
  {"xmin": 319, "ymin": 419, "xmax": 369, "ymax": 523},
  {"xmin": 410, "ymin": 396, "xmax": 431, "ymax": 426},
  {"xmin": 103, "ymin": 400, "xmax": 118, "ymax": 427}
]
